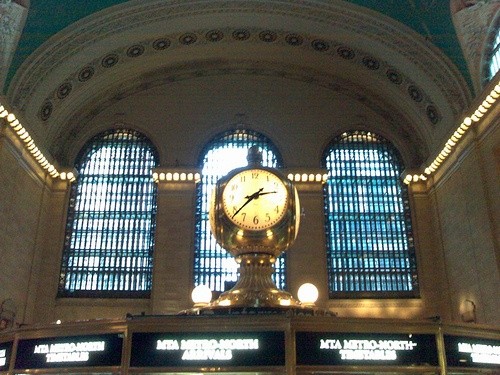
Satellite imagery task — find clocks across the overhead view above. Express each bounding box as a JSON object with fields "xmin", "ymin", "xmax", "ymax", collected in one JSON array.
[{"xmin": 178, "ymin": 145, "xmax": 336, "ymax": 314}]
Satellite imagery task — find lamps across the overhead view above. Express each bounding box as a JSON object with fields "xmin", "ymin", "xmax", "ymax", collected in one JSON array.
[
  {"xmin": 191, "ymin": 284, "xmax": 213, "ymax": 307},
  {"xmin": 297, "ymin": 282, "xmax": 320, "ymax": 308},
  {"xmin": 457, "ymin": 296, "xmax": 475, "ymax": 323}
]
